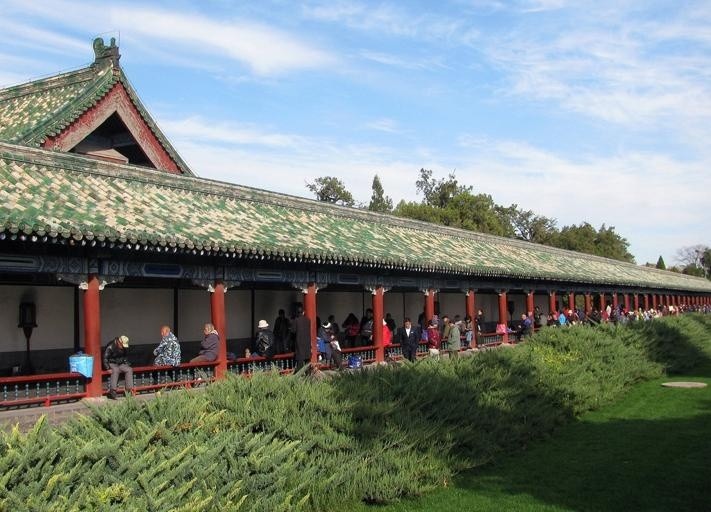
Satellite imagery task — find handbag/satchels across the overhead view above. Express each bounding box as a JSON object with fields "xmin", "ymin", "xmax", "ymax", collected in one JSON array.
[
  {"xmin": 347, "ymin": 354, "xmax": 363, "ymax": 369},
  {"xmin": 329, "ymin": 337, "xmax": 342, "ymax": 352},
  {"xmin": 316, "ymin": 337, "xmax": 326, "ymax": 353},
  {"xmin": 68, "ymin": 348, "xmax": 94, "ymax": 379}
]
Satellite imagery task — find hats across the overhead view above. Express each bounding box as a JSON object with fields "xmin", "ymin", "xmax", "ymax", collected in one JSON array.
[
  {"xmin": 257, "ymin": 319, "xmax": 269, "ymax": 328},
  {"xmin": 119, "ymin": 335, "xmax": 130, "ymax": 348}
]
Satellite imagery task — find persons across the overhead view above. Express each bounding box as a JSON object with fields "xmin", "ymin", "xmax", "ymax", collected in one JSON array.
[
  {"xmin": 190, "ymin": 323, "xmax": 220, "ymax": 363},
  {"xmin": 151, "ymin": 326, "xmax": 182, "ymax": 385},
  {"xmin": 103, "ymin": 335, "xmax": 134, "ymax": 400},
  {"xmin": 513, "ymin": 303, "xmax": 711, "ymax": 341},
  {"xmin": 251, "ymin": 305, "xmax": 487, "ymax": 376}
]
